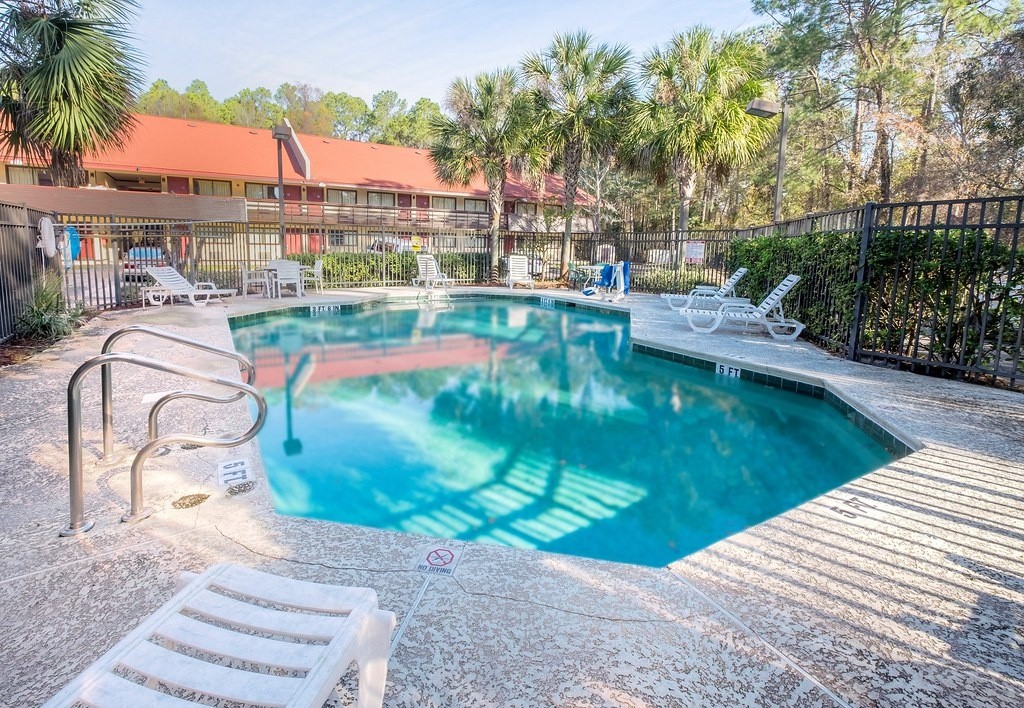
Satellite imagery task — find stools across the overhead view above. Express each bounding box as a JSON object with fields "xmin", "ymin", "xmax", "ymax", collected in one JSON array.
[{"xmin": 717, "ymin": 297, "xmax": 750, "ymax": 307}]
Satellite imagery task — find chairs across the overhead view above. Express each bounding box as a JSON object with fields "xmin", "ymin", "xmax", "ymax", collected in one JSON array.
[
  {"xmin": 567, "ymin": 260, "xmax": 627, "ymax": 303},
  {"xmin": 237, "ymin": 257, "xmax": 326, "ymax": 301},
  {"xmin": 659, "ymin": 267, "xmax": 748, "ymax": 310},
  {"xmin": 507, "ymin": 255, "xmax": 535, "ymax": 291},
  {"xmin": 141, "ymin": 266, "xmax": 238, "ymax": 308},
  {"xmin": 680, "ymin": 275, "xmax": 805, "ymax": 340},
  {"xmin": 411, "ymin": 255, "xmax": 457, "ymax": 287}
]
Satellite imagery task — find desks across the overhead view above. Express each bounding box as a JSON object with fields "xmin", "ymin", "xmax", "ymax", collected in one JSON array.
[
  {"xmin": 579, "ymin": 266, "xmax": 627, "ymax": 299},
  {"xmin": 263, "ymin": 266, "xmax": 308, "ymax": 298}
]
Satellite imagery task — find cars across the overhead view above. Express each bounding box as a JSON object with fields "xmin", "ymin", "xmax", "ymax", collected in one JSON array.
[{"xmin": 121, "ymin": 246, "xmax": 170, "ymax": 281}]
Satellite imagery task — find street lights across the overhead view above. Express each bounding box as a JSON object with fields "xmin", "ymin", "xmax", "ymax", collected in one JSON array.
[
  {"xmin": 271, "ymin": 125, "xmax": 294, "ymax": 290},
  {"xmin": 745, "ymin": 97, "xmax": 790, "ymax": 298}
]
